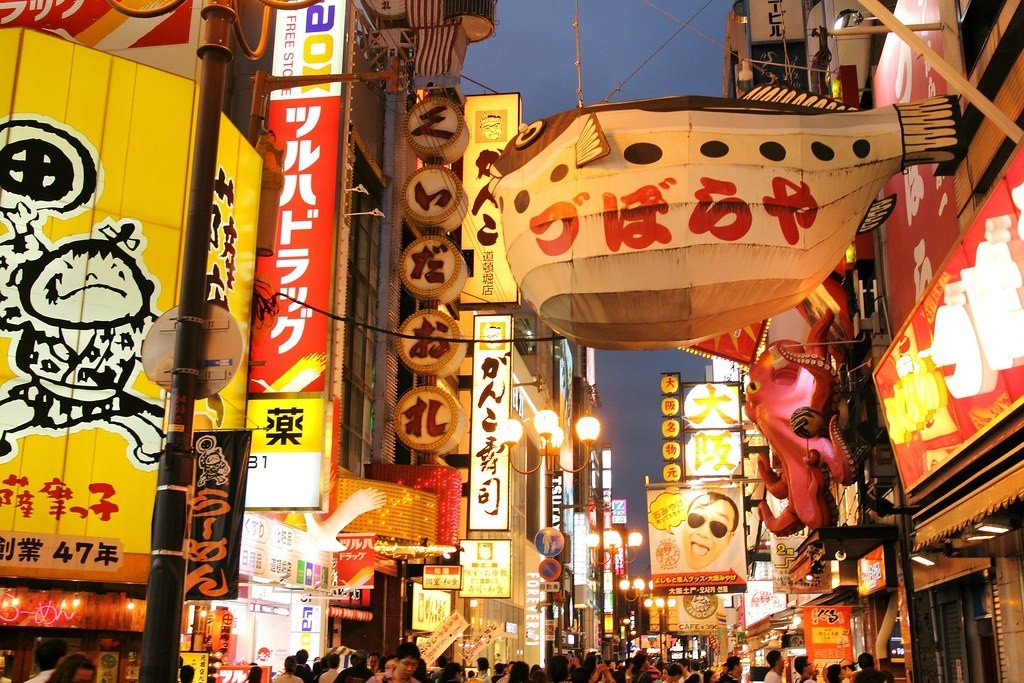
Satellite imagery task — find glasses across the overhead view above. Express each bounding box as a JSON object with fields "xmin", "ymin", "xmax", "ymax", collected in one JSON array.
[{"xmin": 689, "ymin": 512, "xmax": 729, "ymax": 539}]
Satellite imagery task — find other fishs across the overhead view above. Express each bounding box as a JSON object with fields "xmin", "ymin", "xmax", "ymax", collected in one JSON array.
[{"xmin": 488, "ymin": 80, "xmax": 971, "ymax": 353}]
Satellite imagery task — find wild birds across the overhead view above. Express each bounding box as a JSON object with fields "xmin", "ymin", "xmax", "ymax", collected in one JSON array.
[{"xmin": 302, "ymin": 485, "xmax": 389, "ymax": 554}]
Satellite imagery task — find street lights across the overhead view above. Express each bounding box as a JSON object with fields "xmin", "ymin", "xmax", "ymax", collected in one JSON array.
[
  {"xmin": 587, "ymin": 529, "xmax": 643, "ymax": 668},
  {"xmin": 644, "ymin": 597, "xmax": 677, "ymax": 662},
  {"xmin": 497, "ymin": 410, "xmax": 602, "ymax": 683},
  {"xmin": 620, "ymin": 577, "xmax": 654, "ymax": 653}
]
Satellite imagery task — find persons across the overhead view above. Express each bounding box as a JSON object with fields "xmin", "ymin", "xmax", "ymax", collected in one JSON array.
[
  {"xmin": 179, "ymin": 634, "xmax": 898, "ymax": 683},
  {"xmin": 22, "ymin": 639, "xmax": 68, "ymax": 683},
  {"xmin": 47, "ymin": 652, "xmax": 97, "ymax": 683},
  {"xmin": 684, "ymin": 492, "xmax": 738, "ymax": 570}
]
